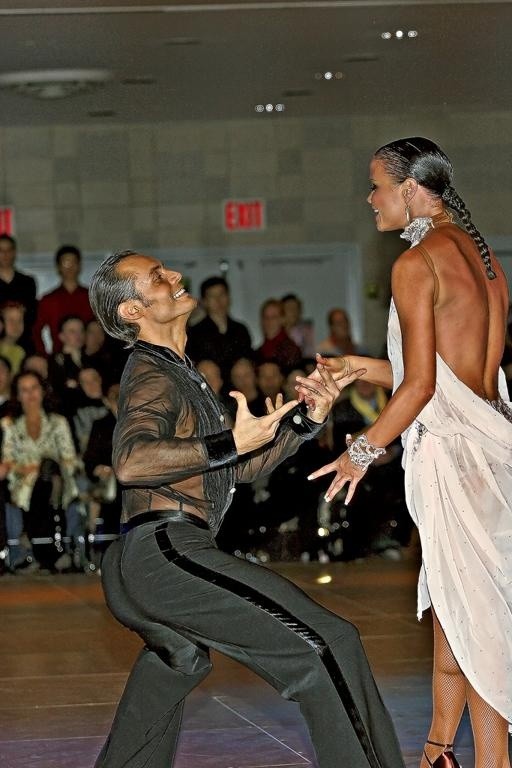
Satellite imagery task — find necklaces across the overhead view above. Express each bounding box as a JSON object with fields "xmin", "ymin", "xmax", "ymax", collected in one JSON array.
[
  {"xmin": 131, "ymin": 343, "xmax": 205, "ymax": 386},
  {"xmin": 400, "ymin": 209, "xmax": 451, "ymax": 246}
]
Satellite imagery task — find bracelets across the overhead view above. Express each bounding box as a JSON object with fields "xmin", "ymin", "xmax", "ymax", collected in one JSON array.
[{"xmin": 342, "ymin": 432, "xmax": 388, "ymax": 470}]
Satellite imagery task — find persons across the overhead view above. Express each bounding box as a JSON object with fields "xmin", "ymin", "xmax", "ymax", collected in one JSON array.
[{"xmin": 87, "ymin": 242, "xmax": 411, "ymax": 768}]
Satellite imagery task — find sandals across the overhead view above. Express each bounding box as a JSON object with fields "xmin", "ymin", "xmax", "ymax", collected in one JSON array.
[{"xmin": 424, "ymin": 740, "xmax": 460, "ymax": 768}]
